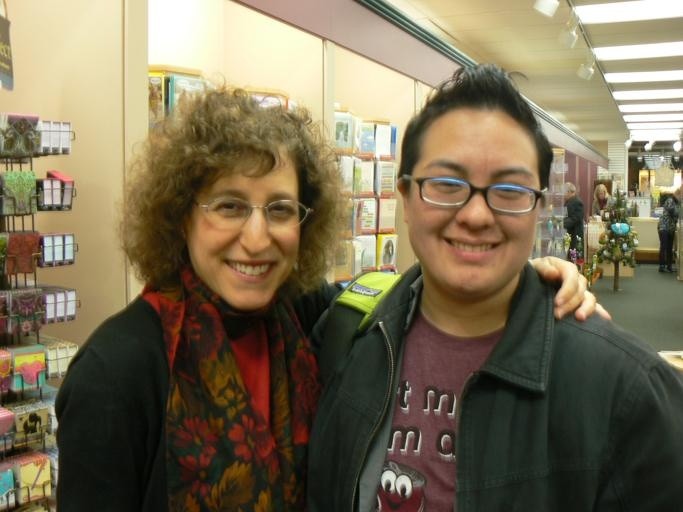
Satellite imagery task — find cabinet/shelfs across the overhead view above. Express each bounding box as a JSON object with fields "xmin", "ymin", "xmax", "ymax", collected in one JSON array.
[
  {"xmin": 531, "ymin": 148, "xmax": 565, "ymax": 258},
  {"xmin": 0, "ymin": 129, "xmax": 81, "ymax": 512}
]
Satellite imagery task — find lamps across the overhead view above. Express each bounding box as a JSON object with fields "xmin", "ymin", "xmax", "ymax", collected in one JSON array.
[
  {"xmin": 534, "ymin": 0, "xmax": 559, "ymax": 17},
  {"xmin": 577, "ymin": 53, "xmax": 596, "ymax": 81},
  {"xmin": 562, "ymin": 17, "xmax": 581, "ymax": 47}
]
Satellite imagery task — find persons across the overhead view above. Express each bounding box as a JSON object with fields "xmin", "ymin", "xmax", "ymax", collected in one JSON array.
[
  {"xmin": 591, "ymin": 184, "xmax": 614, "ymax": 217},
  {"xmin": 306, "ymin": 64, "xmax": 682, "ymax": 510},
  {"xmin": 56, "ymin": 84, "xmax": 611, "ymax": 511},
  {"xmin": 563, "ymin": 182, "xmax": 584, "ymax": 249},
  {"xmin": 658, "ymin": 192, "xmax": 679, "ymax": 273}
]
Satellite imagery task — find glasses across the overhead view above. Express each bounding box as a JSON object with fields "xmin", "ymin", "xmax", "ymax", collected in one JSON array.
[
  {"xmin": 193, "ymin": 196, "xmax": 313, "ymax": 231},
  {"xmin": 400, "ymin": 173, "xmax": 547, "ymax": 214}
]
